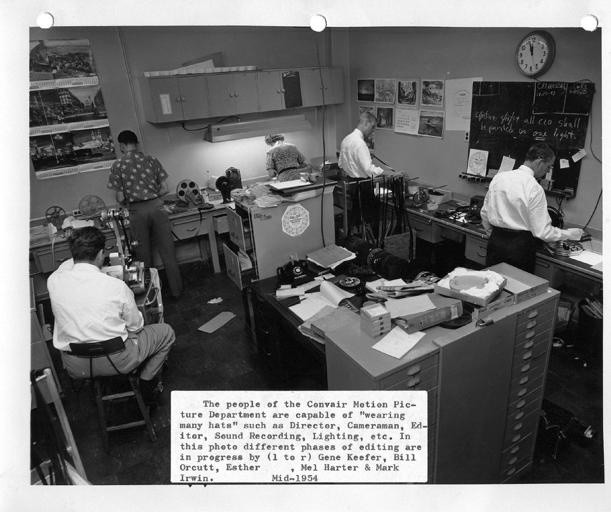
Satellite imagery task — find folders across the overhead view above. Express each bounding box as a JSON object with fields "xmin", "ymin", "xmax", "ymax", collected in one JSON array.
[
  {"xmin": 304, "ymin": 243, "xmax": 356, "ymax": 270},
  {"xmin": 389, "ymin": 292, "xmax": 464, "ymax": 333}
]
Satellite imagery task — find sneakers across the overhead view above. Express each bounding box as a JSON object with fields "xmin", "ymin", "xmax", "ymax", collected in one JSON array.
[{"xmin": 141, "ymin": 382, "xmax": 153, "ymax": 400}]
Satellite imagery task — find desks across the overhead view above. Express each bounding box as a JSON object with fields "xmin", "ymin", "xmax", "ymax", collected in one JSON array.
[
  {"xmin": 222, "ymin": 175, "xmax": 339, "ymax": 292},
  {"xmin": 251, "ymin": 243, "xmax": 435, "ymax": 390},
  {"xmin": 30, "ymin": 231, "xmax": 124, "ymax": 303},
  {"xmin": 127, "ymin": 211, "xmax": 221, "ymax": 288},
  {"xmin": 532, "ymin": 253, "xmax": 603, "ymax": 332},
  {"xmin": 377, "ymin": 203, "xmax": 488, "ymax": 269},
  {"xmin": 199, "ymin": 176, "xmax": 274, "ymax": 274}
]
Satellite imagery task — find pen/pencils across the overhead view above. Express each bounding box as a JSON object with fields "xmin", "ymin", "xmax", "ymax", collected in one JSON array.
[{"xmin": 288, "ymin": 296, "xmax": 307, "ymax": 307}]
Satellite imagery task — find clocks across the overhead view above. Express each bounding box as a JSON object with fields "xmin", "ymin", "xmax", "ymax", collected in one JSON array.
[{"xmin": 514, "ymin": 30, "xmax": 556, "ymax": 78}]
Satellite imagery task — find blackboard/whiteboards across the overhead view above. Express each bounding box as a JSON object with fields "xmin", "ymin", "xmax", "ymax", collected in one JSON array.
[{"xmin": 467, "ymin": 80, "xmax": 595, "ymax": 199}]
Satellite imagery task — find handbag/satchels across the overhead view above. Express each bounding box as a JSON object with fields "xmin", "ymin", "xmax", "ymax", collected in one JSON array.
[{"xmin": 536, "ymin": 410, "xmax": 576, "ymax": 472}]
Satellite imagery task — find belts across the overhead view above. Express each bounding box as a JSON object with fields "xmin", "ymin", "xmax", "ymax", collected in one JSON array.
[{"xmin": 62, "ymin": 351, "xmax": 74, "ymax": 356}]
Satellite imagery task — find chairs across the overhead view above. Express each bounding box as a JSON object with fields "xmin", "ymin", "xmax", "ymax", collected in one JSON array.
[{"xmin": 63, "ymin": 336, "xmax": 158, "ymax": 453}]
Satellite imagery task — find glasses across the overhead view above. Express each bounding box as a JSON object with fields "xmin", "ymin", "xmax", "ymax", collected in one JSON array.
[{"xmin": 546, "ymin": 165, "xmax": 554, "ymax": 169}]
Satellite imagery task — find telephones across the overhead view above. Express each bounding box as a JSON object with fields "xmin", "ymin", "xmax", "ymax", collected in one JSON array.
[{"xmin": 277, "ymin": 259, "xmax": 317, "ymax": 289}]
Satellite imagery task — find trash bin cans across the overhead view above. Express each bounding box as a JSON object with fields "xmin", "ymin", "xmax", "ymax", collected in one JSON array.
[{"xmin": 576, "ymin": 294, "xmax": 603, "ymax": 355}]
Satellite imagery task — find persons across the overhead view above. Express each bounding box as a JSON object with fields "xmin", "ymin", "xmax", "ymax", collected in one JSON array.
[
  {"xmin": 337, "ymin": 112, "xmax": 385, "ymax": 237},
  {"xmin": 480, "ymin": 141, "xmax": 584, "ymax": 273},
  {"xmin": 107, "ymin": 131, "xmax": 183, "ymax": 299},
  {"xmin": 265, "ymin": 132, "xmax": 311, "ymax": 179},
  {"xmin": 46, "ymin": 225, "xmax": 176, "ymax": 406}
]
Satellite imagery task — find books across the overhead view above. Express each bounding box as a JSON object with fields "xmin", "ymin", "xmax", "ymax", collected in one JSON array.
[{"xmin": 437, "ymin": 267, "xmax": 506, "ymax": 308}]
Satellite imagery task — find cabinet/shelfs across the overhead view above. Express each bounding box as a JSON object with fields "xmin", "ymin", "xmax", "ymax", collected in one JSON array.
[
  {"xmin": 206, "ymin": 70, "xmax": 258, "ymax": 118},
  {"xmin": 324, "ymin": 266, "xmax": 560, "ymax": 483},
  {"xmin": 137, "ymin": 74, "xmax": 210, "ymax": 125},
  {"xmin": 258, "ymin": 68, "xmax": 304, "ymax": 112},
  {"xmin": 300, "ymin": 66, "xmax": 345, "ymax": 108}
]
